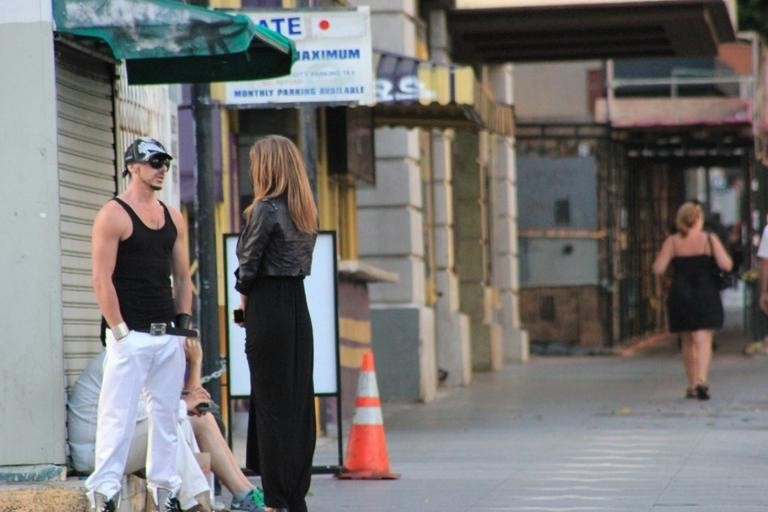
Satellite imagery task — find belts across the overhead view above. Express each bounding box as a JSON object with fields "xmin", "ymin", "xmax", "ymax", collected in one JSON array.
[{"xmin": 106, "ymin": 321, "xmax": 199, "ymax": 337}]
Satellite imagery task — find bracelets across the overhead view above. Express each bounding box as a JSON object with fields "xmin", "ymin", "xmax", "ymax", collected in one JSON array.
[
  {"xmin": 109, "ymin": 321, "xmax": 130, "ymax": 341},
  {"xmin": 174, "ymin": 313, "xmax": 194, "ymax": 330}
]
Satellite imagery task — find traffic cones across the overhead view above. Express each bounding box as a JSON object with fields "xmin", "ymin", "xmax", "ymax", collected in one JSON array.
[{"xmin": 335, "ymin": 353, "xmax": 402, "ymax": 482}]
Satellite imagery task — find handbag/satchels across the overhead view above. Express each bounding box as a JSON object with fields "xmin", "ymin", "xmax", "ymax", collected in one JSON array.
[{"xmin": 718, "ymin": 269, "xmax": 734, "ymax": 290}]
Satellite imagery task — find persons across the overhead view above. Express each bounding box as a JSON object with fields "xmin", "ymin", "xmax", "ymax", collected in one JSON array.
[
  {"xmin": 234, "ymin": 135, "xmax": 319, "ymax": 511},
  {"xmin": 69, "ymin": 314, "xmax": 215, "ymax": 512},
  {"xmin": 756, "ymin": 225, "xmax": 768, "ymax": 315},
  {"xmin": 83, "ymin": 137, "xmax": 193, "ymax": 512},
  {"xmin": 181, "ymin": 337, "xmax": 266, "ymax": 512},
  {"xmin": 651, "ymin": 201, "xmax": 734, "ymax": 400}
]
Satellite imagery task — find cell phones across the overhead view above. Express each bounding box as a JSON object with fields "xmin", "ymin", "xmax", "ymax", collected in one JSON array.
[
  {"xmin": 197, "ymin": 403, "xmax": 210, "ymax": 412},
  {"xmin": 234, "ymin": 310, "xmax": 246, "ymax": 322}
]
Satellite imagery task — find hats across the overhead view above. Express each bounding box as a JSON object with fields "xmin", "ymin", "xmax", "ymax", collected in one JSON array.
[{"xmin": 124, "ymin": 137, "xmax": 172, "ymax": 163}]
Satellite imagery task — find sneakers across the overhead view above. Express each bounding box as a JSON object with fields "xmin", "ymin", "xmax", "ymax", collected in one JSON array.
[{"xmin": 230, "ymin": 487, "xmax": 266, "ymax": 512}]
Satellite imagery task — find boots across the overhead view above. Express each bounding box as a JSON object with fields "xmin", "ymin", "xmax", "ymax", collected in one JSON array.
[
  {"xmin": 94, "ymin": 491, "xmax": 120, "ymax": 512},
  {"xmin": 148, "ymin": 487, "xmax": 184, "ymax": 512}
]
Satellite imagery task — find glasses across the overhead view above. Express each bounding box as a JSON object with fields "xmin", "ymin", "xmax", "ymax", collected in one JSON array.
[{"xmin": 149, "ymin": 156, "xmax": 171, "ymax": 169}]
